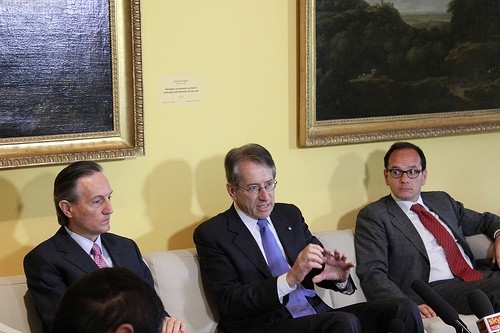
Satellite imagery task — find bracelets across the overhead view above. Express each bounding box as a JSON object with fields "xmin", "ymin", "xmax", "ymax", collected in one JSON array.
[{"xmin": 495, "ymin": 231, "xmax": 500, "ymax": 239}]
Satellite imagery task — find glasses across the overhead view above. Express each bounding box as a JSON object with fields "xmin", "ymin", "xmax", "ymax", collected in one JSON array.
[
  {"xmin": 237, "ymin": 178, "xmax": 278, "ymax": 196},
  {"xmin": 385, "ymin": 168, "xmax": 424, "ymax": 179}
]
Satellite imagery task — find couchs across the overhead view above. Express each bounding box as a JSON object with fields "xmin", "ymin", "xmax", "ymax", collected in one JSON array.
[{"xmin": 0, "ymin": 228, "xmax": 500, "ymax": 333}]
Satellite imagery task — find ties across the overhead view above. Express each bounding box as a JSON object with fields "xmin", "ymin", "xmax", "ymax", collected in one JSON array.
[
  {"xmin": 90, "ymin": 243, "xmax": 109, "ymax": 269},
  {"xmin": 410, "ymin": 203, "xmax": 485, "ymax": 281},
  {"xmin": 257, "ymin": 218, "xmax": 316, "ymax": 318}
]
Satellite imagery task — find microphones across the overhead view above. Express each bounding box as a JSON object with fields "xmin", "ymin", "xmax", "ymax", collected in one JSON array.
[
  {"xmin": 410, "ymin": 279, "xmax": 471, "ymax": 333},
  {"xmin": 467, "ymin": 288, "xmax": 500, "ymax": 333}
]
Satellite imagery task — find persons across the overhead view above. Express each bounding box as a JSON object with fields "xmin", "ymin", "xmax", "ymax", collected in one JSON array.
[
  {"xmin": 192, "ymin": 144, "xmax": 425, "ymax": 333},
  {"xmin": 23, "ymin": 161, "xmax": 187, "ymax": 333},
  {"xmin": 356, "ymin": 142, "xmax": 500, "ymax": 318},
  {"xmin": 49, "ymin": 267, "xmax": 164, "ymax": 333}
]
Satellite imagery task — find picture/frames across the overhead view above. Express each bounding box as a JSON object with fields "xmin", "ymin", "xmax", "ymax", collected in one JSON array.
[
  {"xmin": 296, "ymin": 0, "xmax": 500, "ymax": 149},
  {"xmin": 0, "ymin": 0, "xmax": 145, "ymax": 170}
]
